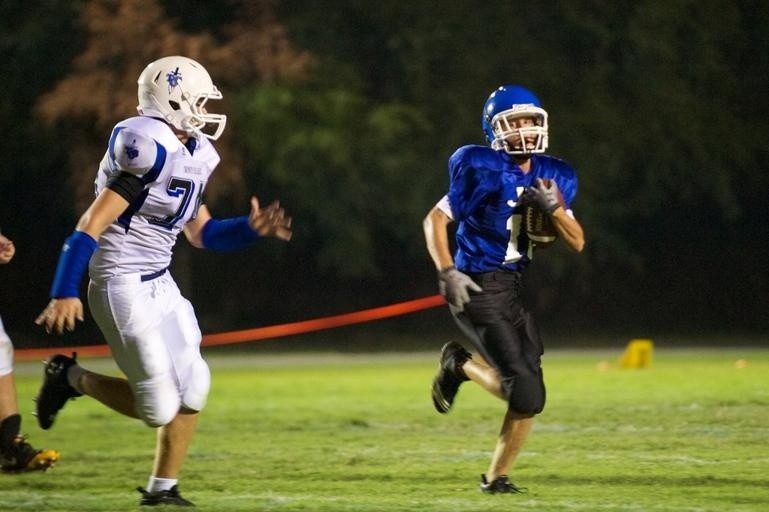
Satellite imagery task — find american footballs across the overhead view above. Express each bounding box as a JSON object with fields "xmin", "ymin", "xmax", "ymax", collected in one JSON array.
[{"xmin": 521, "ymin": 180, "xmax": 566, "ymax": 250}]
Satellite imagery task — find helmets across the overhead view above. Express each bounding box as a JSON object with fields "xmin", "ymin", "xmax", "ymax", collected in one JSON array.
[
  {"xmin": 136, "ymin": 55, "xmax": 224, "ymax": 132},
  {"xmin": 481, "ymin": 83, "xmax": 543, "ymax": 146}
]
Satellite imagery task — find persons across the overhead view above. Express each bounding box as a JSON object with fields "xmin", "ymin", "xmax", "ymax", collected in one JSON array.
[
  {"xmin": 421, "ymin": 84, "xmax": 584, "ymax": 497},
  {"xmin": 33, "ymin": 55, "xmax": 293, "ymax": 508},
  {"xmin": 0, "ymin": 234, "xmax": 62, "ymax": 476}
]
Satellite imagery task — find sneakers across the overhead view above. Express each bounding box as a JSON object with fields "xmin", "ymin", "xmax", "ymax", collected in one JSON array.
[
  {"xmin": 431, "ymin": 340, "xmax": 472, "ymax": 413},
  {"xmin": 135, "ymin": 485, "xmax": 195, "ymax": 505},
  {"xmin": 480, "ymin": 473, "xmax": 527, "ymax": 495},
  {"xmin": 0, "ymin": 433, "xmax": 60, "ymax": 474},
  {"xmin": 35, "ymin": 352, "xmax": 83, "ymax": 430}
]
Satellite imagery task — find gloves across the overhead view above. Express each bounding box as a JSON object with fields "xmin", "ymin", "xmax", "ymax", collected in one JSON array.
[
  {"xmin": 527, "ymin": 177, "xmax": 563, "ymax": 216},
  {"xmin": 437, "ymin": 265, "xmax": 483, "ymax": 310}
]
